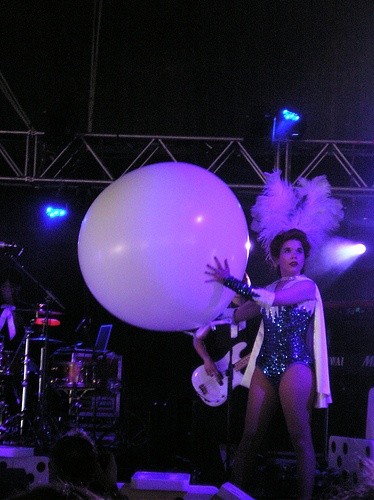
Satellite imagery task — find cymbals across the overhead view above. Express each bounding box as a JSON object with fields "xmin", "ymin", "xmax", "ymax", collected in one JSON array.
[
  {"xmin": 13, "ymin": 309, "xmax": 61, "ymax": 317},
  {"xmin": 30, "ymin": 337, "xmax": 63, "ymax": 344}
]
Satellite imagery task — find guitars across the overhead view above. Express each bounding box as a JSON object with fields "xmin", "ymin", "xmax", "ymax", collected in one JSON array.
[{"xmin": 192, "ymin": 341, "xmax": 252, "ymax": 407}]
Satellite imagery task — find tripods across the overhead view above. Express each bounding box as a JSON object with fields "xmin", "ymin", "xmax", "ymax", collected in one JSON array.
[{"xmin": 0, "ymin": 250, "xmax": 66, "ymax": 447}]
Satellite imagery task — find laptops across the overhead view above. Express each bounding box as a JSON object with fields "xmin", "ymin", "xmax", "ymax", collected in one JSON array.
[{"xmin": 76, "ymin": 324, "xmax": 114, "ymax": 353}]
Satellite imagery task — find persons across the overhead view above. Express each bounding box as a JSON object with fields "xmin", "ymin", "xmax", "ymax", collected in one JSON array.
[
  {"xmin": 0, "ymin": 426, "xmax": 374, "ymax": 500},
  {"xmin": 193, "ymin": 273, "xmax": 262, "ymax": 487},
  {"xmin": 204, "ymin": 229, "xmax": 333, "ymax": 500}
]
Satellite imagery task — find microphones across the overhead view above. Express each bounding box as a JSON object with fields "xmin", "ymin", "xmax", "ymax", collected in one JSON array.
[{"xmin": 0, "ymin": 241, "xmax": 18, "ymax": 249}]
